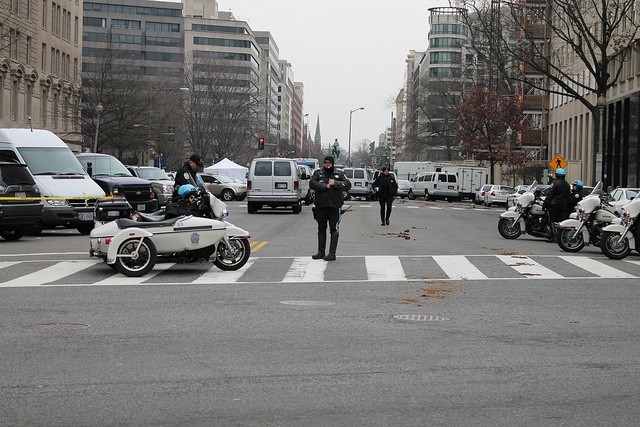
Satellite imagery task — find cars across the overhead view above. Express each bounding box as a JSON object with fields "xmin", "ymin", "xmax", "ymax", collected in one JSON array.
[
  {"xmin": 506, "ymin": 183, "xmax": 531, "ymax": 210},
  {"xmin": 393, "ymin": 180, "xmax": 411, "ymax": 200},
  {"xmin": 474, "ymin": 184, "xmax": 491, "ymax": 204},
  {"xmin": 611, "ymin": 184, "xmax": 640, "ymax": 208},
  {"xmin": 76, "ymin": 150, "xmax": 160, "ymax": 213},
  {"xmin": 483, "ymin": 185, "xmax": 507, "ymax": 206},
  {"xmin": 131, "ymin": 159, "xmax": 180, "ymax": 209}
]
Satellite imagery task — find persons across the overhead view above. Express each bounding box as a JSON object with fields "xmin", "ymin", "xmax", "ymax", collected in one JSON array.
[
  {"xmin": 172, "ymin": 153, "xmax": 205, "ymax": 204},
  {"xmin": 308, "ymin": 156, "xmax": 352, "ymax": 261},
  {"xmin": 331, "ymin": 138, "xmax": 341, "ymax": 159},
  {"xmin": 569, "ymin": 180, "xmax": 588, "ymax": 206},
  {"xmin": 541, "ymin": 168, "xmax": 573, "ymax": 242},
  {"xmin": 373, "ymin": 166, "xmax": 399, "ymax": 226}
]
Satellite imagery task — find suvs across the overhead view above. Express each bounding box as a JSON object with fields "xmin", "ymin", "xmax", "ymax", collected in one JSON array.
[
  {"xmin": 197, "ymin": 174, "xmax": 246, "ymax": 200},
  {"xmin": 0, "ymin": 161, "xmax": 44, "ymax": 240}
]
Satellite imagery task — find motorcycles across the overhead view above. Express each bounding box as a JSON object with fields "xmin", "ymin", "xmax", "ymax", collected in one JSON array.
[
  {"xmin": 601, "ymin": 189, "xmax": 640, "ymax": 259},
  {"xmin": 89, "ymin": 183, "xmax": 252, "ymax": 278},
  {"xmin": 560, "ymin": 185, "xmax": 631, "ymax": 252},
  {"xmin": 496, "ymin": 182, "xmax": 582, "ymax": 240}
]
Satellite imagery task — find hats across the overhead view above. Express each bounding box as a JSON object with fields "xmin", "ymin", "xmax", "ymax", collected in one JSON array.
[
  {"xmin": 189, "ymin": 154, "xmax": 204, "ymax": 168},
  {"xmin": 324, "ymin": 155, "xmax": 334, "ymax": 162},
  {"xmin": 382, "ymin": 167, "xmax": 389, "ymax": 171}
]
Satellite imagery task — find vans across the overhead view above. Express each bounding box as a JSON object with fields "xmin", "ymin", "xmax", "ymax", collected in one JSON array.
[
  {"xmin": 343, "ymin": 166, "xmax": 373, "ymax": 200},
  {"xmin": 408, "ymin": 170, "xmax": 460, "ymax": 201},
  {"xmin": 0, "ymin": 127, "xmax": 105, "ymax": 236},
  {"xmin": 297, "ymin": 153, "xmax": 324, "ymax": 206},
  {"xmin": 247, "ymin": 157, "xmax": 303, "ymax": 214}
]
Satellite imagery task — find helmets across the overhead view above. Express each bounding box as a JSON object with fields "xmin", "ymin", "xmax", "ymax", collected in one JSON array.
[
  {"xmin": 571, "ymin": 179, "xmax": 583, "ymax": 188},
  {"xmin": 555, "ymin": 168, "xmax": 566, "ymax": 177},
  {"xmin": 178, "ymin": 184, "xmax": 197, "ymax": 198}
]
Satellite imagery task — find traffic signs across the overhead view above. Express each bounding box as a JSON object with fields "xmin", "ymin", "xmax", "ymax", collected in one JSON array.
[{"xmin": 550, "ymin": 155, "xmax": 567, "ymax": 180}]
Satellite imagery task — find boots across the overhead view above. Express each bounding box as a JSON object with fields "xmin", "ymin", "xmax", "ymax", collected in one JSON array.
[
  {"xmin": 323, "ymin": 231, "xmax": 340, "ymax": 261},
  {"xmin": 386, "ymin": 210, "xmax": 393, "ymax": 225},
  {"xmin": 312, "ymin": 232, "xmax": 326, "ymax": 259},
  {"xmin": 380, "ymin": 210, "xmax": 386, "ymax": 226},
  {"xmin": 546, "ymin": 222, "xmax": 557, "ymax": 244}
]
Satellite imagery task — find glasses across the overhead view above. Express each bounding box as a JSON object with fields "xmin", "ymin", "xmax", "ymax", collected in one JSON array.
[{"xmin": 323, "ymin": 161, "xmax": 333, "ymax": 164}]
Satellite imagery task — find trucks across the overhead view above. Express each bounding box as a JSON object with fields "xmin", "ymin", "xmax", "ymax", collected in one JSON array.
[
  {"xmin": 440, "ymin": 167, "xmax": 490, "ymax": 200},
  {"xmin": 393, "ymin": 161, "xmax": 457, "ymax": 180}
]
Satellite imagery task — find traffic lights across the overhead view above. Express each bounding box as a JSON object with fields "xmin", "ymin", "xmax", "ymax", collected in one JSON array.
[{"xmin": 260, "ymin": 138, "xmax": 266, "ymax": 149}]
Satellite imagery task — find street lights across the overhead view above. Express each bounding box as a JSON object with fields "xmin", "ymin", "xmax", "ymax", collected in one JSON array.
[
  {"xmin": 347, "ymin": 106, "xmax": 365, "ymax": 167},
  {"xmin": 140, "ymin": 84, "xmax": 192, "ymax": 164},
  {"xmin": 597, "ymin": 92, "xmax": 610, "ymax": 188},
  {"xmin": 91, "ymin": 102, "xmax": 103, "ymax": 152}
]
